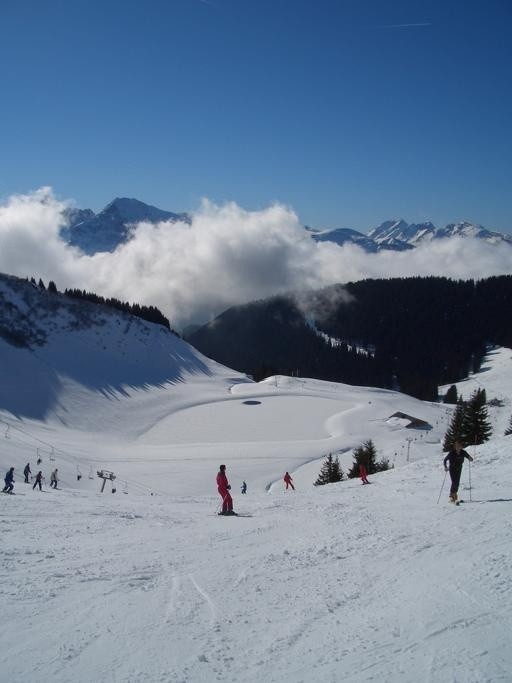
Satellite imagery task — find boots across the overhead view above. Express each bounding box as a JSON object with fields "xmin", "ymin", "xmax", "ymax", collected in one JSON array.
[
  {"xmin": 449, "ymin": 493, "xmax": 458, "ymax": 502},
  {"xmin": 221, "ymin": 510, "xmax": 234, "ymax": 515},
  {"xmin": 363, "ymin": 481, "xmax": 370, "ymax": 484}
]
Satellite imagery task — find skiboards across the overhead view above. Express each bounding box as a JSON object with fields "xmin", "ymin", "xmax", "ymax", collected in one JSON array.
[
  {"xmin": 216, "ymin": 511, "xmax": 253, "ymax": 519},
  {"xmin": 449, "ymin": 495, "xmax": 466, "ymax": 508}
]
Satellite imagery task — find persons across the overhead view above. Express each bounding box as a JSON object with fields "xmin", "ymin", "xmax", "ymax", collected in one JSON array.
[
  {"xmin": 283, "ymin": 472, "xmax": 295, "ymax": 490},
  {"xmin": 215, "ymin": 464, "xmax": 237, "ymax": 516},
  {"xmin": 240, "ymin": 480, "xmax": 247, "ymax": 495},
  {"xmin": 443, "ymin": 439, "xmax": 474, "ymax": 502},
  {"xmin": 1, "ymin": 462, "xmax": 58, "ymax": 494},
  {"xmin": 357, "ymin": 461, "xmax": 368, "ymax": 485}
]
